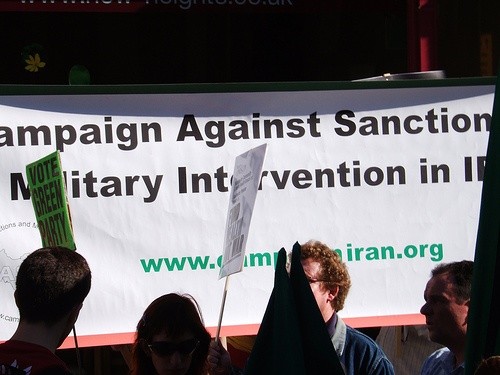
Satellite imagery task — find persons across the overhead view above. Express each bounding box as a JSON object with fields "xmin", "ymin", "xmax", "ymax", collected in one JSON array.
[
  {"xmin": 206, "ymin": 242, "xmax": 397, "ymax": 375},
  {"xmin": 420, "ymin": 260, "xmax": 476, "ymax": 375},
  {"xmin": 131, "ymin": 292, "xmax": 212, "ymax": 375},
  {"xmin": 0, "ymin": 246, "xmax": 92, "ymax": 375}
]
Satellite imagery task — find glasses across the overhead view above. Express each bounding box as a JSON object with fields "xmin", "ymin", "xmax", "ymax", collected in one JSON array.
[{"xmin": 146, "ymin": 336, "xmax": 199, "ymax": 357}]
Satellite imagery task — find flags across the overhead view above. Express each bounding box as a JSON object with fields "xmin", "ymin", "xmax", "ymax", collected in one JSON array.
[{"xmin": 464, "ymin": 60, "xmax": 500, "ymax": 375}]
